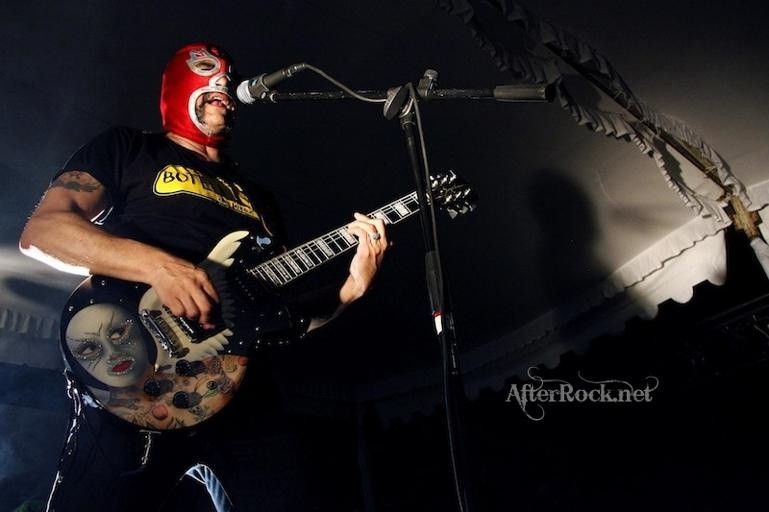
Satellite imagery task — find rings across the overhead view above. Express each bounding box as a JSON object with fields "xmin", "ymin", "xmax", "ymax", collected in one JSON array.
[{"xmin": 370, "ymin": 234, "xmax": 380, "ymax": 240}]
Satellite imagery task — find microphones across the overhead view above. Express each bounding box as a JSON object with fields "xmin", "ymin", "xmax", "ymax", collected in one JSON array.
[{"xmin": 236, "ymin": 63, "xmax": 304, "ymax": 104}]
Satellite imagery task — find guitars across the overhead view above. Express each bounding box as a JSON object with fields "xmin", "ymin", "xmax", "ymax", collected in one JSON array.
[{"xmin": 58, "ymin": 169, "xmax": 477, "ymax": 431}]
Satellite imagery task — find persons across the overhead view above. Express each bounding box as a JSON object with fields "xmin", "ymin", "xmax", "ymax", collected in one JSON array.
[
  {"xmin": 18, "ymin": 42, "xmax": 393, "ymax": 512},
  {"xmin": 65, "ymin": 302, "xmax": 249, "ymax": 431}
]
